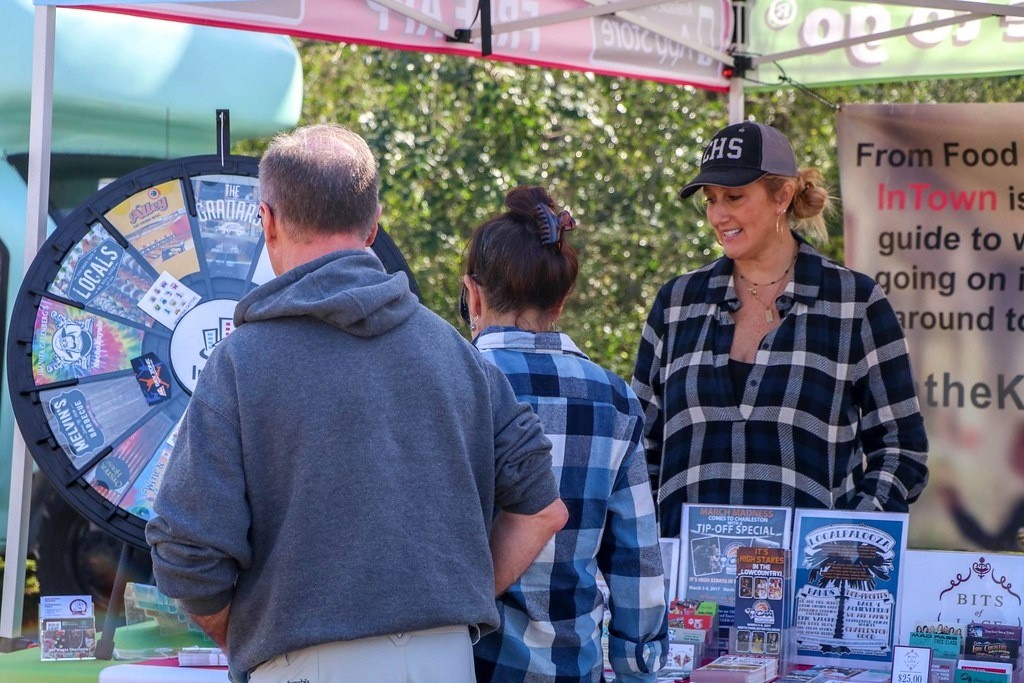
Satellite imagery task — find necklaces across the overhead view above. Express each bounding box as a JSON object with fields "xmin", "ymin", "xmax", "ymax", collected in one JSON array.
[{"xmin": 732, "ymin": 253, "xmax": 800, "ymax": 323}]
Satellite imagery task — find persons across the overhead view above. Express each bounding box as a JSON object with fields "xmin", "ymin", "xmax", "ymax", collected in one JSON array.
[
  {"xmin": 452, "ymin": 187, "xmax": 667, "ymax": 683},
  {"xmin": 143, "ymin": 121, "xmax": 568, "ymax": 683},
  {"xmin": 630, "ymin": 123, "xmax": 929, "ymax": 537}
]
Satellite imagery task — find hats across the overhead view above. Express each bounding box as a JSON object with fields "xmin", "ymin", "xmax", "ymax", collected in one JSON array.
[{"xmin": 678, "ymin": 122, "xmax": 799, "ymax": 199}]
